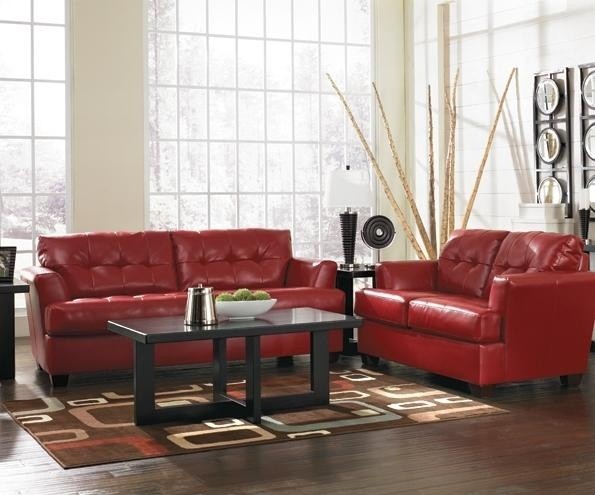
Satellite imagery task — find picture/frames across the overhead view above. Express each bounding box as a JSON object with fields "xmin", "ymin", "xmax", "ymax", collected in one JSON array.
[{"xmin": 0, "ymin": 247, "xmax": 16, "ymax": 284}]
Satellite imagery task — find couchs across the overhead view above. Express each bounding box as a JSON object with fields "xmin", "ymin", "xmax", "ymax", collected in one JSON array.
[
  {"xmin": 21, "ymin": 228, "xmax": 345, "ymax": 387},
  {"xmin": 355, "ymin": 230, "xmax": 595, "ymax": 398}
]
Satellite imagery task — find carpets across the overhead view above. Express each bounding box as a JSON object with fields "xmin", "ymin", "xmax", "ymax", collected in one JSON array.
[{"xmin": 1, "ymin": 368, "xmax": 512, "ymax": 470}]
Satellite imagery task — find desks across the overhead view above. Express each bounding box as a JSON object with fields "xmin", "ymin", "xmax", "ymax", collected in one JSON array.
[
  {"xmin": 0, "ymin": 276, "xmax": 29, "ymax": 380},
  {"xmin": 336, "ymin": 264, "xmax": 376, "ymax": 356}
]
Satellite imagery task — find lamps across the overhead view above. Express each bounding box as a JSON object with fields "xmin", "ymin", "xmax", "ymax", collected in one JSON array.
[{"xmin": 322, "ymin": 165, "xmax": 375, "ymax": 269}]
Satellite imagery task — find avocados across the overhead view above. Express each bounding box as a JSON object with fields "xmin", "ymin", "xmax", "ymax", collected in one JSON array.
[{"xmin": 215, "ymin": 288, "xmax": 271, "ymax": 300}]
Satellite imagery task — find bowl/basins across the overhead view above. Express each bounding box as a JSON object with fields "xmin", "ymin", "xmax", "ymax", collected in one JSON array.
[{"xmin": 214, "ymin": 300, "xmax": 277, "ymax": 320}]
